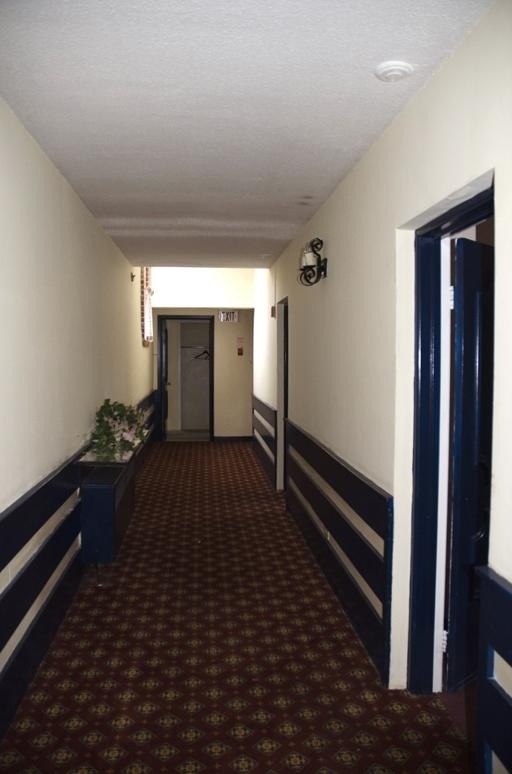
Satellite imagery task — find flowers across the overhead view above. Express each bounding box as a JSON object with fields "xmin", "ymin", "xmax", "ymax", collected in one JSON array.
[{"xmin": 85, "ymin": 397, "xmax": 147, "ymax": 463}]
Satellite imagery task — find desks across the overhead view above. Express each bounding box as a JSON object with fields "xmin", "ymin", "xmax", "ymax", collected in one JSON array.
[{"xmin": 73, "ymin": 431, "xmax": 150, "ymax": 564}]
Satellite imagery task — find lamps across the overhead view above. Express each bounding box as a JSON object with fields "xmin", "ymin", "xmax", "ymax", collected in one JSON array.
[{"xmin": 295, "ymin": 236, "xmax": 327, "ymax": 288}]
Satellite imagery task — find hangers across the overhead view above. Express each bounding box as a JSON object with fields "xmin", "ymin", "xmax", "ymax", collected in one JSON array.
[{"xmin": 193, "ymin": 350, "xmax": 211, "ymax": 361}]
